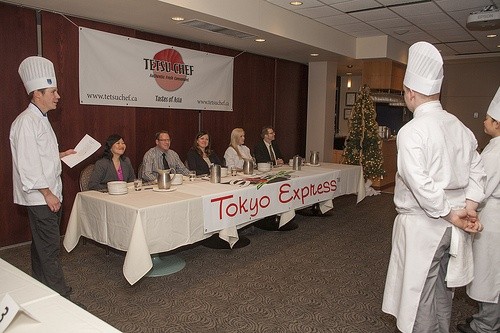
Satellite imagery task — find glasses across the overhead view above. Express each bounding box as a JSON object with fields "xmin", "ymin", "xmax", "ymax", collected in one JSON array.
[
  {"xmin": 159, "ymin": 139, "xmax": 171, "ymax": 142},
  {"xmin": 268, "ymin": 131, "xmax": 275, "ymax": 135}
]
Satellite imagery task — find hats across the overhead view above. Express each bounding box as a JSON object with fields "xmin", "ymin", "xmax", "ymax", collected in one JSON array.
[
  {"xmin": 17, "ymin": 56, "xmax": 57, "ymax": 95},
  {"xmin": 487, "ymin": 86, "xmax": 500, "ymax": 122},
  {"xmin": 403, "ymin": 41, "xmax": 444, "ymax": 96}
]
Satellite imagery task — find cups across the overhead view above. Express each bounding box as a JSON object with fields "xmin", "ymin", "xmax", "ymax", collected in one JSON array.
[
  {"xmin": 267, "ymin": 161, "xmax": 273, "ymax": 170},
  {"xmin": 133, "ymin": 178, "xmax": 142, "ymax": 192},
  {"xmin": 231, "ymin": 166, "xmax": 238, "ymax": 176},
  {"xmin": 301, "ymin": 158, "xmax": 306, "ymax": 166},
  {"xmin": 188, "ymin": 170, "xmax": 196, "ymax": 182}
]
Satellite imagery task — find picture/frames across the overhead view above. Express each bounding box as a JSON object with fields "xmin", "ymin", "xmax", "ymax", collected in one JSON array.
[
  {"xmin": 346, "ymin": 92, "xmax": 356, "ymax": 105},
  {"xmin": 344, "ymin": 108, "xmax": 352, "ymax": 119}
]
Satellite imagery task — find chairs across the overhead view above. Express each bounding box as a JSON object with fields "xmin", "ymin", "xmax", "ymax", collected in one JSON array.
[{"xmin": 79, "ymin": 164, "xmax": 96, "ymax": 191}]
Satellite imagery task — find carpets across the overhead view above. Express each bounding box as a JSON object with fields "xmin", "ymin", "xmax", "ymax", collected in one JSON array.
[{"xmin": 0, "ymin": 190, "xmax": 480, "ymax": 333}]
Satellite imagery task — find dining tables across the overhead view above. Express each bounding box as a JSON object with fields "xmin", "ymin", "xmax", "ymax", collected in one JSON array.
[{"xmin": 63, "ymin": 160, "xmax": 368, "ymax": 286}]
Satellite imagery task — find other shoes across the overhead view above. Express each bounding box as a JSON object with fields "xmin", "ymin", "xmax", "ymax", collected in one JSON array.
[
  {"xmin": 465, "ymin": 317, "xmax": 473, "ymax": 323},
  {"xmin": 456, "ymin": 324, "xmax": 475, "ymax": 333},
  {"xmin": 63, "ymin": 297, "xmax": 87, "ymax": 310},
  {"xmin": 63, "ymin": 286, "xmax": 72, "ymax": 294}
]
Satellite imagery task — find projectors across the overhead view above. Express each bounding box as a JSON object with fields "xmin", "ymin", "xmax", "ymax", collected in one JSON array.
[{"xmin": 465, "ymin": 11, "xmax": 500, "ymax": 32}]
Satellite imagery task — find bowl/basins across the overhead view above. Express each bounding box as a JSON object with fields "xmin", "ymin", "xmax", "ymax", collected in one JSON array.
[
  {"xmin": 257, "ymin": 162, "xmax": 271, "ymax": 172},
  {"xmin": 169, "ymin": 173, "xmax": 183, "ymax": 185},
  {"xmin": 107, "ymin": 181, "xmax": 127, "ymax": 194},
  {"xmin": 221, "ymin": 167, "xmax": 228, "ymax": 178}
]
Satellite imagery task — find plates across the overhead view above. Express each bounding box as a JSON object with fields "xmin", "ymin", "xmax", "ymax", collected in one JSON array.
[
  {"xmin": 153, "ymin": 186, "xmax": 177, "ymax": 191},
  {"xmin": 241, "ymin": 172, "xmax": 257, "ymax": 176},
  {"xmin": 308, "ymin": 163, "xmax": 320, "ymax": 166}
]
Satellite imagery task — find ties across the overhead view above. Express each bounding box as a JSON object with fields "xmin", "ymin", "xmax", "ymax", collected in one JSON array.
[
  {"xmin": 161, "ymin": 153, "xmax": 170, "ymax": 170},
  {"xmin": 269, "ymin": 144, "xmax": 276, "ymax": 165}
]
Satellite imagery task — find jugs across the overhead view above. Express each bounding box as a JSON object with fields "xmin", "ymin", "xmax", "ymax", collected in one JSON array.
[
  {"xmin": 292, "ymin": 155, "xmax": 302, "ymax": 171},
  {"xmin": 209, "ymin": 163, "xmax": 222, "ymax": 184},
  {"xmin": 242, "ymin": 157, "xmax": 258, "ymax": 175},
  {"xmin": 383, "ymin": 127, "xmax": 392, "ymax": 139},
  {"xmin": 156, "ymin": 167, "xmax": 176, "ymax": 190},
  {"xmin": 310, "ymin": 151, "xmax": 320, "ymax": 165}
]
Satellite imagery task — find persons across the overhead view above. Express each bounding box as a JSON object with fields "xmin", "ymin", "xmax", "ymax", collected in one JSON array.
[
  {"xmin": 456, "ymin": 104, "xmax": 500, "ymax": 333},
  {"xmin": 88, "ymin": 134, "xmax": 137, "ymax": 192},
  {"xmin": 223, "ymin": 128, "xmax": 257, "ymax": 170},
  {"xmin": 394, "ymin": 63, "xmax": 487, "ymax": 333},
  {"xmin": 191, "ymin": 129, "xmax": 227, "ymax": 175},
  {"xmin": 10, "ymin": 75, "xmax": 87, "ymax": 310},
  {"xmin": 139, "ymin": 130, "xmax": 193, "ymax": 184},
  {"xmin": 256, "ymin": 127, "xmax": 285, "ymax": 166}
]
